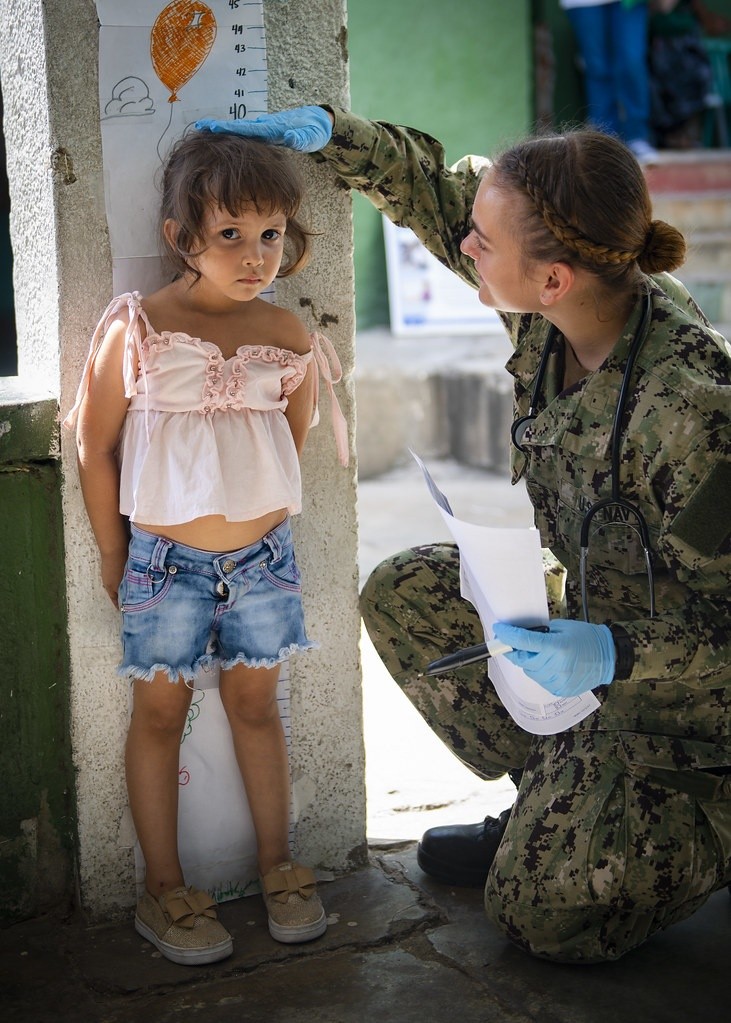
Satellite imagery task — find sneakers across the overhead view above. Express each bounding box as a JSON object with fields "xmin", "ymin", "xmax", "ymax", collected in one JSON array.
[
  {"xmin": 134, "ymin": 887, "xmax": 234, "ymax": 965},
  {"xmin": 417, "ymin": 804, "xmax": 514, "ymax": 890},
  {"xmin": 257, "ymin": 857, "xmax": 326, "ymax": 942}
]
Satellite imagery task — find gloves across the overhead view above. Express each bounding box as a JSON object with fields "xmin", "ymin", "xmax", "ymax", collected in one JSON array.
[
  {"xmin": 194, "ymin": 106, "xmax": 333, "ymax": 152},
  {"xmin": 492, "ymin": 619, "xmax": 615, "ymax": 698}
]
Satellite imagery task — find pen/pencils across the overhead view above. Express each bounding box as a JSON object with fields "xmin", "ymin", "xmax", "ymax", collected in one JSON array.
[{"xmin": 416, "ymin": 624, "xmax": 548, "ymax": 680}]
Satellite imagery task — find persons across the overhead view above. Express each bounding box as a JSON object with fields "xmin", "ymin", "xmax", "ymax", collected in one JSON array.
[
  {"xmin": 559, "ymin": 0, "xmax": 664, "ymax": 168},
  {"xmin": 75, "ymin": 129, "xmax": 326, "ymax": 967},
  {"xmin": 195, "ymin": 104, "xmax": 731, "ymax": 964}
]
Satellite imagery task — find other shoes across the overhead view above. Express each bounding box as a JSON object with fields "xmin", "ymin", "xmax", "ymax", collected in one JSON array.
[{"xmin": 629, "ymin": 141, "xmax": 659, "ymax": 164}]
